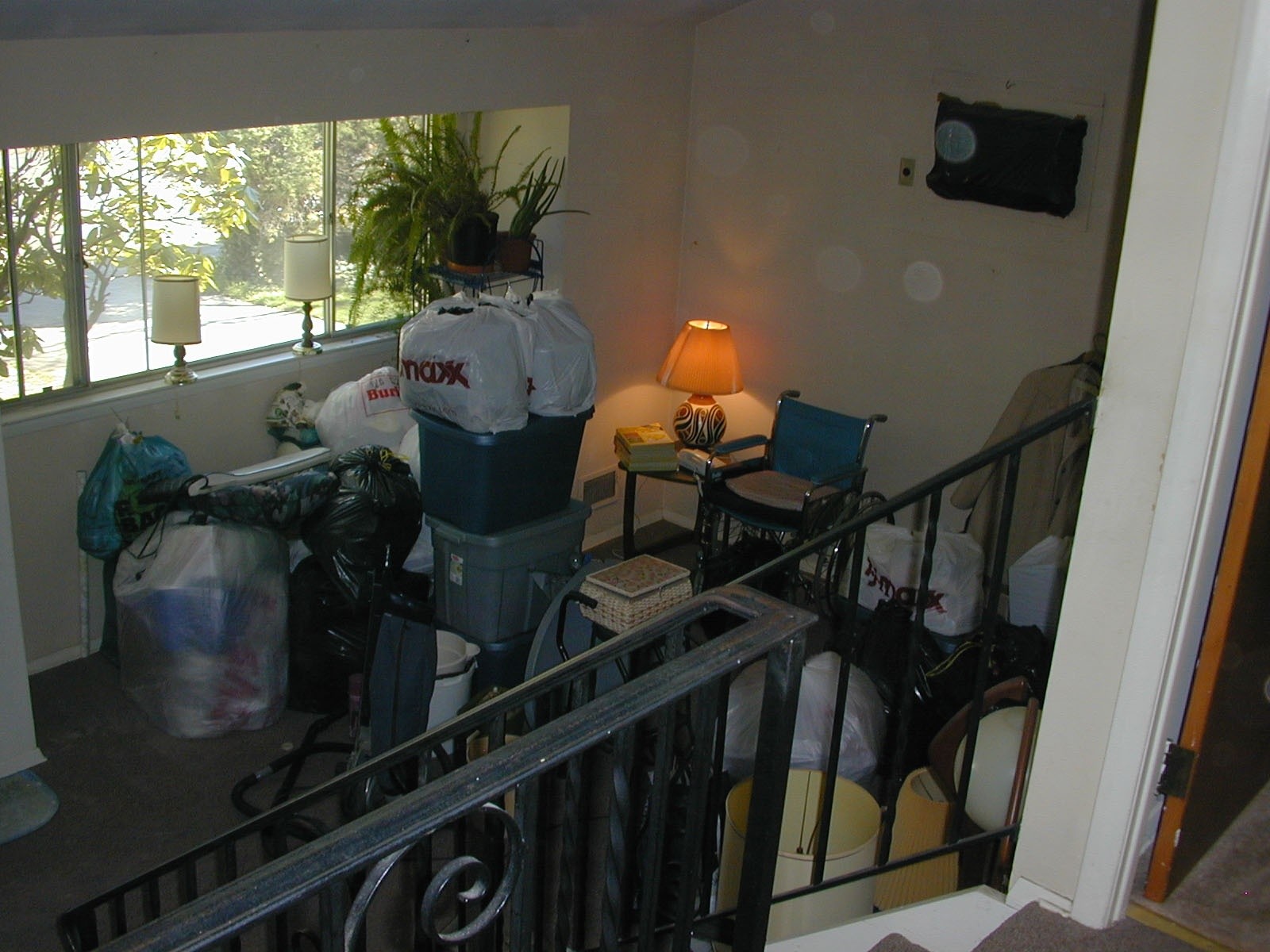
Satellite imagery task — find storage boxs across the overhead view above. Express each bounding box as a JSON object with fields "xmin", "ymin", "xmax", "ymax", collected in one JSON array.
[
  {"xmin": 407, "ymin": 406, "xmax": 596, "ymax": 536},
  {"xmin": 431, "ymin": 614, "xmax": 539, "ymax": 699},
  {"xmin": 425, "ymin": 499, "xmax": 592, "ymax": 644}
]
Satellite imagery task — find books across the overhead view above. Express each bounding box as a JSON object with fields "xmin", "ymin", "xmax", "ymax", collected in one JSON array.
[{"xmin": 614, "ymin": 421, "xmax": 678, "ymax": 472}]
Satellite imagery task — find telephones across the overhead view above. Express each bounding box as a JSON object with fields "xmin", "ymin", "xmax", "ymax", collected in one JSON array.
[{"xmin": 677, "ymin": 448, "xmax": 725, "ymax": 476}]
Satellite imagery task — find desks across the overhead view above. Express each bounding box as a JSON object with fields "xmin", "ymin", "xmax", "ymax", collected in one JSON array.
[{"xmin": 618, "ymin": 440, "xmax": 738, "ymax": 578}]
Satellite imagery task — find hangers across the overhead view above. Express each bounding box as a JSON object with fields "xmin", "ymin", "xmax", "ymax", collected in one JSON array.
[{"xmin": 1072, "ymin": 332, "xmax": 1108, "ymax": 395}]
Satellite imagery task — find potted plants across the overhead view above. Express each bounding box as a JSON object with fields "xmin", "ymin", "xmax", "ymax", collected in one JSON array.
[
  {"xmin": 338, "ymin": 111, "xmax": 562, "ymax": 327},
  {"xmin": 496, "ymin": 157, "xmax": 590, "ymax": 274}
]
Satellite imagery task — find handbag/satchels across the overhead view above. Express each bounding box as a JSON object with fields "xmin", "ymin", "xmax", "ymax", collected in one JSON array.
[
  {"xmin": 1008, "ymin": 533, "xmax": 1074, "ymax": 638},
  {"xmin": 285, "ymin": 443, "xmax": 437, "ymax": 716},
  {"xmin": 75, "ymin": 423, "xmax": 191, "ymax": 564},
  {"xmin": 847, "ymin": 520, "xmax": 987, "ymax": 635},
  {"xmin": 399, "ymin": 289, "xmax": 597, "ymax": 434},
  {"xmin": 111, "ymin": 509, "xmax": 293, "ymax": 740},
  {"xmin": 313, "ymin": 364, "xmax": 417, "ymax": 454},
  {"xmin": 398, "ymin": 424, "xmax": 436, "ymax": 574},
  {"xmin": 713, "ymin": 649, "xmax": 887, "ymax": 783}
]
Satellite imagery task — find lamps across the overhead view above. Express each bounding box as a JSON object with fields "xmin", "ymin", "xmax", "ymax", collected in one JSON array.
[
  {"xmin": 151, "ymin": 275, "xmax": 202, "ymax": 385},
  {"xmin": 714, "ymin": 768, "xmax": 883, "ymax": 952},
  {"xmin": 656, "ymin": 319, "xmax": 744, "ymax": 450},
  {"xmin": 284, "ymin": 233, "xmax": 332, "ymax": 356},
  {"xmin": 874, "ymin": 767, "xmax": 961, "ymax": 912}
]
